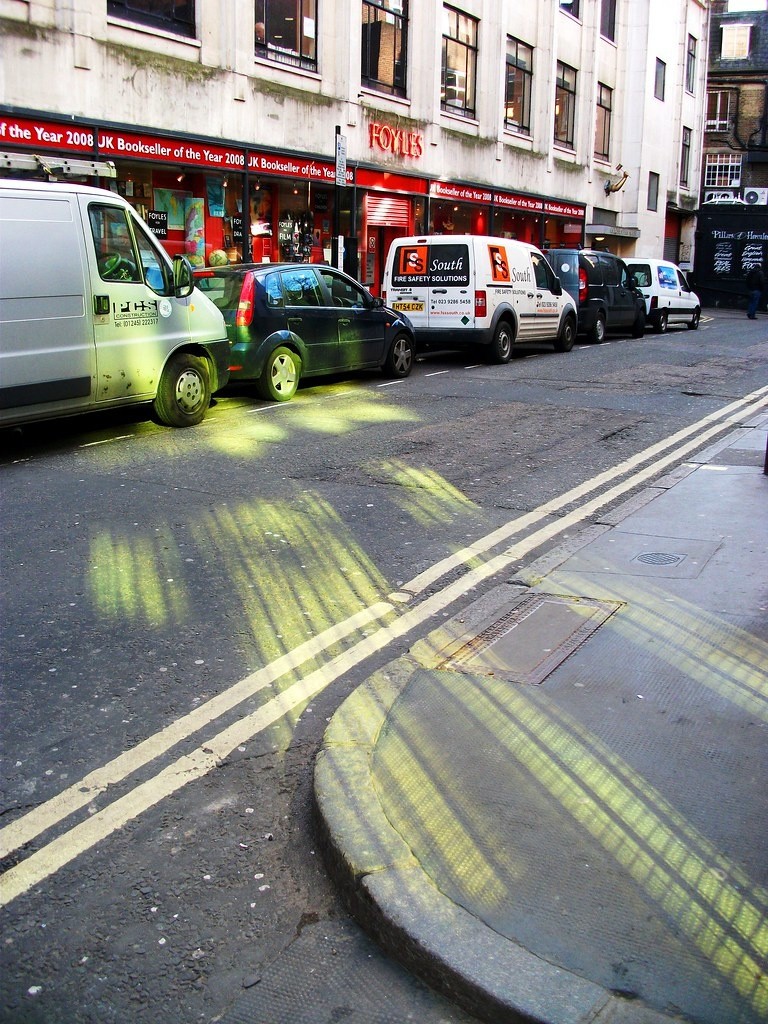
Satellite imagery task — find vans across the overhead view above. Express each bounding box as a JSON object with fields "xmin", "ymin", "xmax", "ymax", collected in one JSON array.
[
  {"xmin": 384, "ymin": 235, "xmax": 578, "ymax": 363},
  {"xmin": 542, "ymin": 248, "xmax": 645, "ymax": 344},
  {"xmin": 620, "ymin": 257, "xmax": 701, "ymax": 333},
  {"xmin": 0, "ymin": 177, "xmax": 229, "ymax": 427}
]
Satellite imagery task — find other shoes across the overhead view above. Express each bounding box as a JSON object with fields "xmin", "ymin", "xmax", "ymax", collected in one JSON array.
[{"xmin": 748, "ymin": 317, "xmax": 758, "ymax": 320}]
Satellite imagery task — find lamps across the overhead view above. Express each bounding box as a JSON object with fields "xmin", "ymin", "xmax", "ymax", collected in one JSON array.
[
  {"xmin": 222, "ymin": 174, "xmax": 228, "ymax": 187},
  {"xmin": 255, "ymin": 179, "xmax": 261, "ymax": 191},
  {"xmin": 177, "ymin": 168, "xmax": 186, "ymax": 182}
]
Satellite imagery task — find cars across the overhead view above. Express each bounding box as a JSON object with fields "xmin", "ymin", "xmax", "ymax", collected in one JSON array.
[{"xmin": 194, "ymin": 262, "xmax": 416, "ymax": 401}]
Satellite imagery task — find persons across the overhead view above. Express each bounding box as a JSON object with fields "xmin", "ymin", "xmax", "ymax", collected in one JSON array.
[{"xmin": 745, "ymin": 264, "xmax": 765, "ymax": 320}]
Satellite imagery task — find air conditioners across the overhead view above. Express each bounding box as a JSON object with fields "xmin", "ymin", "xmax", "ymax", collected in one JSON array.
[{"xmin": 744, "ymin": 186, "xmax": 768, "ymax": 206}]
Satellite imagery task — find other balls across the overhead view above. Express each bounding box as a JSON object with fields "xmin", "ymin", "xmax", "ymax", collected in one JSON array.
[{"xmin": 208, "ymin": 249, "xmax": 228, "ymax": 266}]
